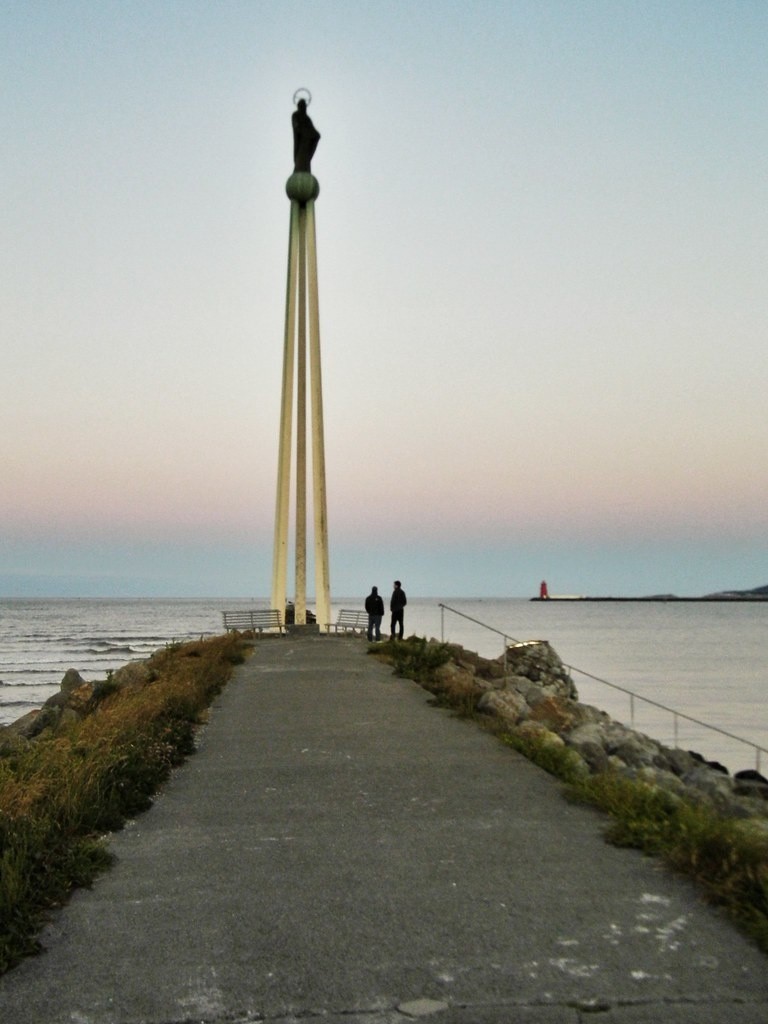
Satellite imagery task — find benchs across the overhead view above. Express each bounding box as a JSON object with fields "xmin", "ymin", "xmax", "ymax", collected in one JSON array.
[
  {"xmin": 221, "ymin": 609, "xmax": 284, "ymax": 640},
  {"xmin": 324, "ymin": 609, "xmax": 369, "ymax": 637}
]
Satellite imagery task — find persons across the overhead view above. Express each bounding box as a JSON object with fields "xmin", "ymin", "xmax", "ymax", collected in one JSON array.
[
  {"xmin": 292, "ymin": 98, "xmax": 321, "ymax": 173},
  {"xmin": 365, "ymin": 586, "xmax": 384, "ymax": 642},
  {"xmin": 390, "ymin": 580, "xmax": 406, "ymax": 640}
]
